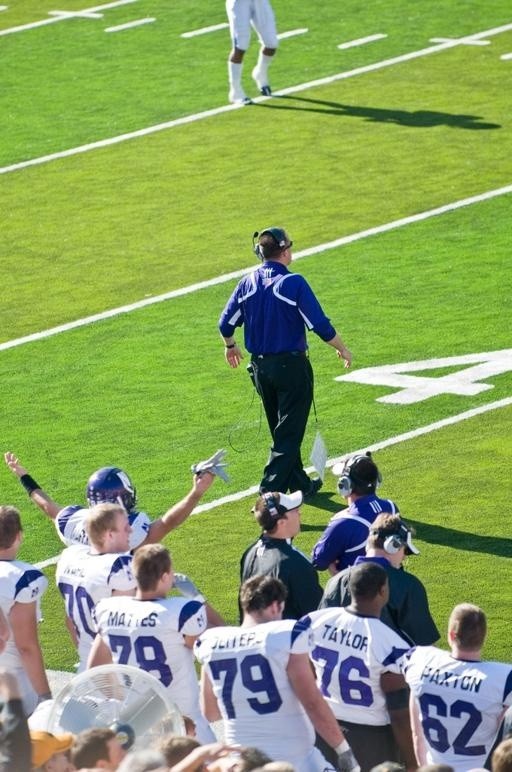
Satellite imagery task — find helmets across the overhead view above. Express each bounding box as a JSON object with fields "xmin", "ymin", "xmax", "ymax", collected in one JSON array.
[{"xmin": 87, "ymin": 467, "xmax": 138, "ymax": 518}]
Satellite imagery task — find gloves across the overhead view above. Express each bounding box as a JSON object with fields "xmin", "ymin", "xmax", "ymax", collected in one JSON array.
[
  {"xmin": 172, "ymin": 573, "xmax": 198, "ymax": 598},
  {"xmin": 191, "ymin": 448, "xmax": 231, "ymax": 484},
  {"xmin": 338, "ymin": 749, "xmax": 362, "ymax": 772}
]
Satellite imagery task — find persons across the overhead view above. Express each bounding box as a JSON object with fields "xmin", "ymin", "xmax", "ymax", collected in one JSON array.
[
  {"xmin": 216, "ymin": 227, "xmax": 354, "ymax": 495},
  {"xmin": 225, "ymin": 0, "xmax": 279, "ymax": 105},
  {"xmin": 0, "ymin": 451, "xmax": 512, "ymax": 772}
]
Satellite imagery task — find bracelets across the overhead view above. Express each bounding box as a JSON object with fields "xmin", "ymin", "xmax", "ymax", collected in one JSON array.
[{"xmin": 225, "ymin": 341, "xmax": 236, "ymax": 349}]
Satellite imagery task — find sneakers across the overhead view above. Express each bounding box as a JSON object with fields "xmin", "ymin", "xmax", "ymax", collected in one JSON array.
[
  {"xmin": 253, "ymin": 67, "xmax": 273, "ymax": 97},
  {"xmin": 229, "ymin": 88, "xmax": 253, "ymax": 106},
  {"xmin": 304, "ymin": 477, "xmax": 323, "ymax": 503}
]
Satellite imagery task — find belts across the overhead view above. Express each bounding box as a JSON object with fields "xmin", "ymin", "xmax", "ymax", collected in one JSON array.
[{"xmin": 291, "ymin": 350, "xmax": 307, "ymax": 357}]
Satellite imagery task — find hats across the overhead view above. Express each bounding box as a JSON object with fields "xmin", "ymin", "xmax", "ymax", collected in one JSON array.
[
  {"xmin": 370, "ymin": 521, "xmax": 421, "ymax": 557},
  {"xmin": 262, "ymin": 490, "xmax": 304, "ymax": 533},
  {"xmin": 332, "ymin": 460, "xmax": 350, "ymax": 476},
  {"xmin": 28, "ymin": 730, "xmax": 74, "ymax": 769}
]
